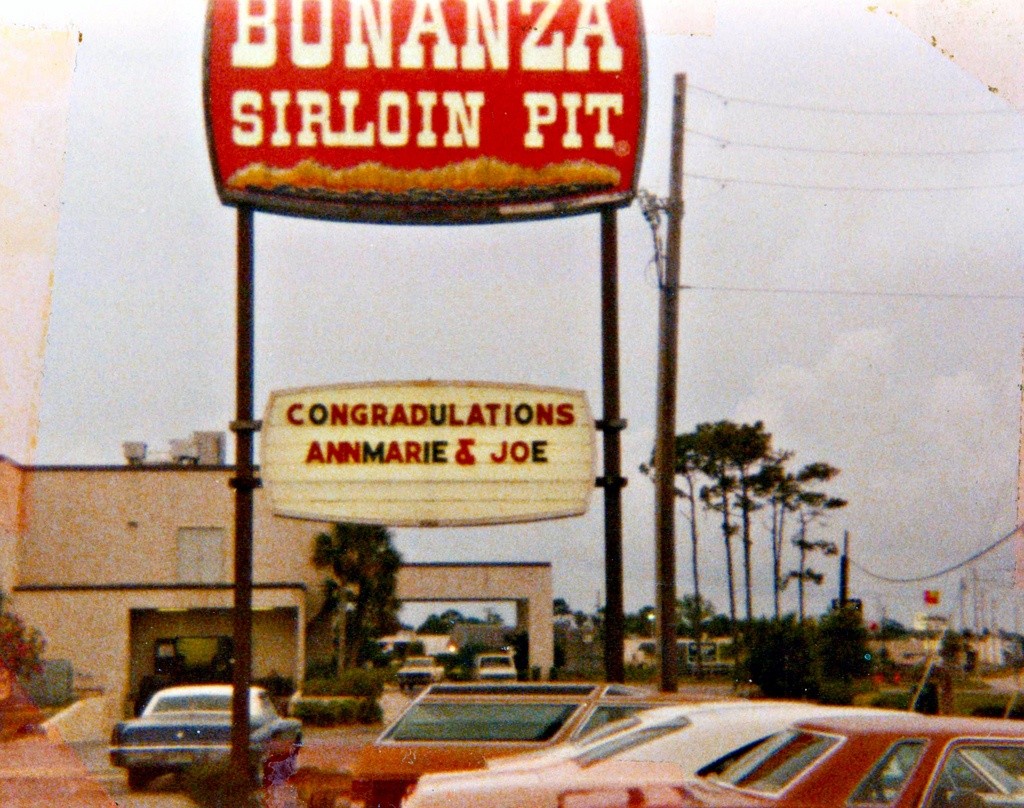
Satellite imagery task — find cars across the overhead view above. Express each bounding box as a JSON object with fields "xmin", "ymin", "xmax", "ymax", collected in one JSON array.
[
  {"xmin": 472, "ymin": 653, "xmax": 517, "ymax": 681},
  {"xmin": 409, "ymin": 701, "xmax": 928, "ymax": 790},
  {"xmin": 405, "ymin": 712, "xmax": 1024, "ymax": 807},
  {"xmin": 395, "ymin": 656, "xmax": 436, "ymax": 691},
  {"xmin": 287, "ymin": 682, "xmax": 747, "ymax": 808},
  {"xmin": 110, "ymin": 682, "xmax": 305, "ymax": 791}
]
